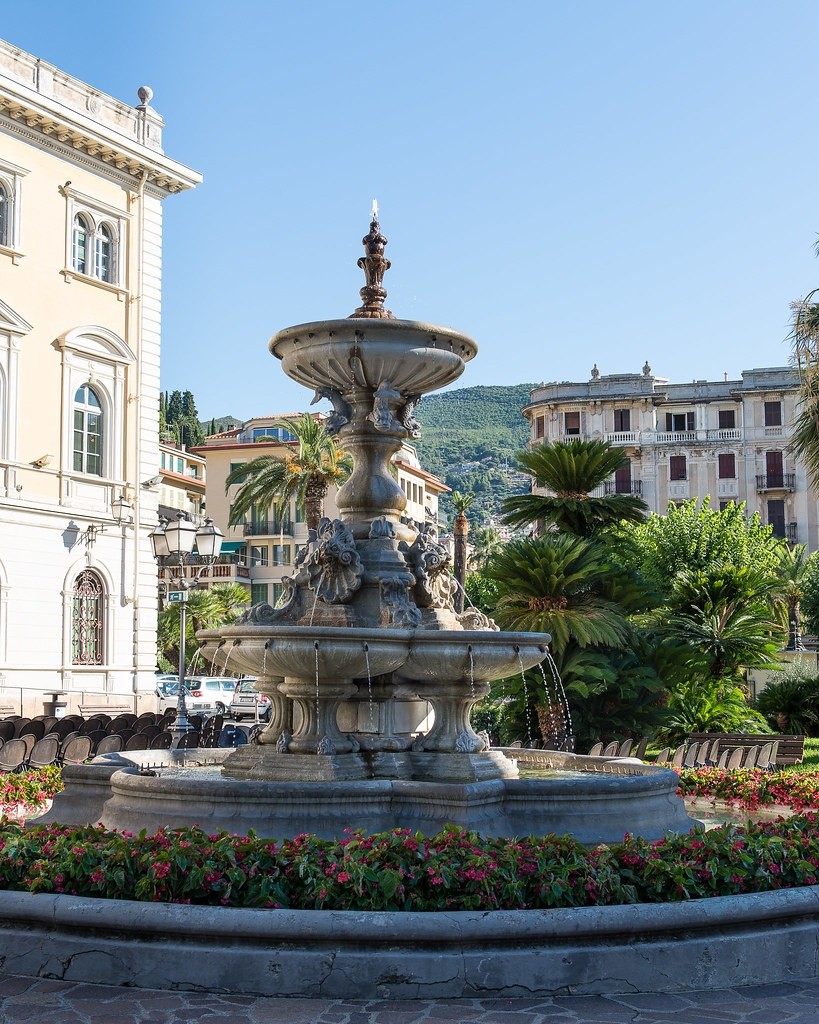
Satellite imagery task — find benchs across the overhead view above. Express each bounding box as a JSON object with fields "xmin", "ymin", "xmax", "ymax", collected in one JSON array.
[{"xmin": 687, "ymin": 733, "xmax": 804, "ymax": 765}]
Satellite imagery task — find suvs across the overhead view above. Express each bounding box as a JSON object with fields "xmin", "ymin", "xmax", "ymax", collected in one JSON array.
[{"xmin": 168, "ymin": 676, "xmax": 239, "ymax": 716}]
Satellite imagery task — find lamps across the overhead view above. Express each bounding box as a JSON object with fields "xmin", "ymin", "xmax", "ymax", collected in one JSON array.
[
  {"xmin": 35, "ymin": 454, "xmax": 54, "ymax": 466},
  {"xmin": 87, "ymin": 495, "xmax": 131, "ymax": 541},
  {"xmin": 142, "ymin": 474, "xmax": 164, "ymax": 485}
]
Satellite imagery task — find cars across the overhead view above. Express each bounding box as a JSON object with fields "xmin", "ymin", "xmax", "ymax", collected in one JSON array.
[
  {"xmin": 157, "ymin": 680, "xmax": 217, "ymax": 717},
  {"xmin": 229, "ymin": 677, "xmax": 273, "ymax": 723},
  {"xmin": 157, "ymin": 674, "xmax": 179, "ymax": 691}
]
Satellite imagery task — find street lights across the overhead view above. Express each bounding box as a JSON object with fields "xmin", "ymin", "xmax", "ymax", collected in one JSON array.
[{"xmin": 147, "ymin": 512, "xmax": 225, "ymax": 747}]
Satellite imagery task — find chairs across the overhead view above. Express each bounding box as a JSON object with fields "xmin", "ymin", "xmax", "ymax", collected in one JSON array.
[{"xmin": 1, "ymin": 707, "xmax": 780, "ymax": 789}]
